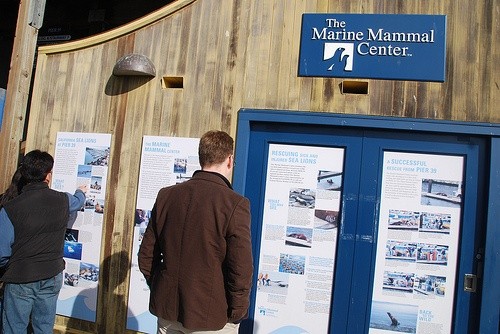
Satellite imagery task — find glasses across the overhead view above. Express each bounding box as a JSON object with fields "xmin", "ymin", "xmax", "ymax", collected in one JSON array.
[{"xmin": 229, "ymin": 155, "xmax": 238, "ymax": 167}]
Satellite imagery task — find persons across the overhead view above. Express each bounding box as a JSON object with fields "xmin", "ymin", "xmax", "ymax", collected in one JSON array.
[
  {"xmin": 137, "ymin": 127, "xmax": 253, "ymax": 334},
  {"xmin": 387, "ymin": 211, "xmax": 447, "ymax": 297},
  {"xmin": 0, "ymin": 164, "xmax": 24, "ymax": 303},
  {"xmin": 0, "ymin": 149, "xmax": 87, "ymax": 334}
]
